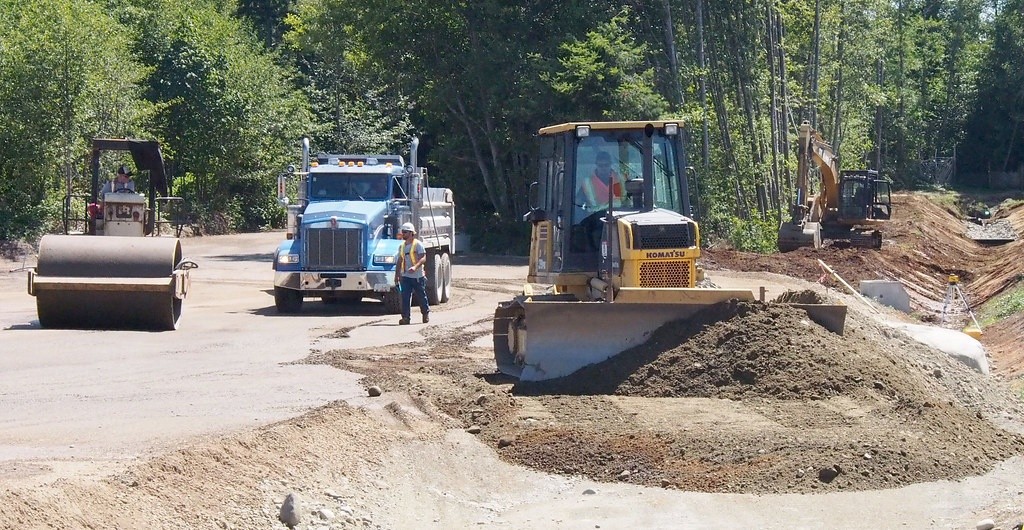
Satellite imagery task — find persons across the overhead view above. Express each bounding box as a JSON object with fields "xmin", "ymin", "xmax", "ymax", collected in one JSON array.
[
  {"xmin": 99, "ymin": 167, "xmax": 134, "ymax": 201},
  {"xmin": 575, "ymin": 152, "xmax": 627, "ymax": 209},
  {"xmin": 394, "ymin": 222, "xmax": 430, "ymax": 324}
]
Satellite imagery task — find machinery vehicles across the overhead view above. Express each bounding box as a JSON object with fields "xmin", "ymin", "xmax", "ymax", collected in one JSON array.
[
  {"xmin": 26, "ymin": 138, "xmax": 199, "ymax": 329},
  {"xmin": 272, "ymin": 136, "xmax": 457, "ymax": 315},
  {"xmin": 776, "ymin": 121, "xmax": 894, "ymax": 253},
  {"xmin": 491, "ymin": 116, "xmax": 848, "ymax": 391}
]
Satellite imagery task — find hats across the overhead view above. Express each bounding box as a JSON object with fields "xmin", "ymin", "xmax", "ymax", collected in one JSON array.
[
  {"xmin": 401, "ymin": 222, "xmax": 416, "ymax": 234},
  {"xmin": 118, "ymin": 167, "xmax": 134, "ymax": 176}
]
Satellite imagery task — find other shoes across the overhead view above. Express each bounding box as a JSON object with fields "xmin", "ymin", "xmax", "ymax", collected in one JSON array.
[
  {"xmin": 399, "ymin": 316, "xmax": 410, "ymax": 325},
  {"xmin": 423, "ymin": 313, "xmax": 429, "ymax": 323}
]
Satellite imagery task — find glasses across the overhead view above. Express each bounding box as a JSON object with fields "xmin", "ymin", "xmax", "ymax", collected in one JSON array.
[
  {"xmin": 595, "ymin": 162, "xmax": 611, "ymax": 168},
  {"xmin": 402, "ymin": 231, "xmax": 409, "ymax": 233}
]
